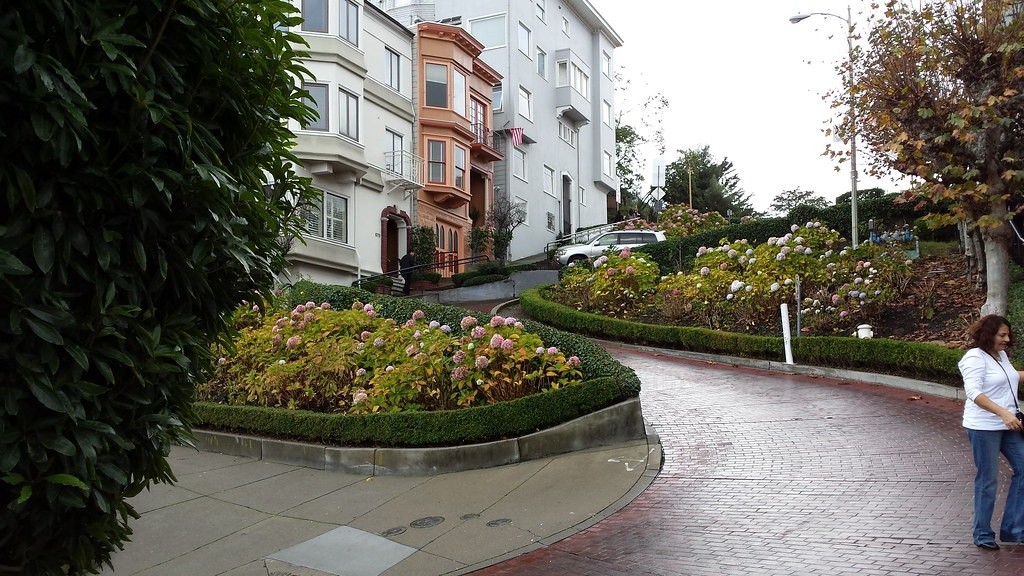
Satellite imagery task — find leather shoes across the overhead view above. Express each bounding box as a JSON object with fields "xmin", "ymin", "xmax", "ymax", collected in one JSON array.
[{"xmin": 979, "ymin": 542, "xmax": 999, "ymax": 550}]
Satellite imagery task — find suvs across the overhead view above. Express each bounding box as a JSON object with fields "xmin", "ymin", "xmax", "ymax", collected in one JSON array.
[{"xmin": 552, "ymin": 229, "xmax": 668, "ymax": 270}]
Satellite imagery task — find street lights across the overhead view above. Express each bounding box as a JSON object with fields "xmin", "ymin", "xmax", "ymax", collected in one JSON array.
[{"xmin": 788, "ymin": 12, "xmax": 859, "ymax": 250}]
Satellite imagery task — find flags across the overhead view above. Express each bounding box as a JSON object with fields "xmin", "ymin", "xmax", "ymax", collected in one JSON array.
[{"xmin": 509, "ymin": 127, "xmax": 524, "ymax": 146}]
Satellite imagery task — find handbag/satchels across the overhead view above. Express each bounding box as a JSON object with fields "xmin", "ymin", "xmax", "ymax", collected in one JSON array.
[{"xmin": 1016, "ymin": 412, "xmax": 1024, "ymax": 426}]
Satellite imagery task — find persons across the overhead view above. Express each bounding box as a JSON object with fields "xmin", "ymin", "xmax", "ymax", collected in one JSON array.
[
  {"xmin": 958, "ymin": 314, "xmax": 1024, "ymax": 551},
  {"xmin": 614, "ymin": 209, "xmax": 640, "ymax": 222},
  {"xmin": 400, "ymin": 249, "xmax": 415, "ymax": 296}
]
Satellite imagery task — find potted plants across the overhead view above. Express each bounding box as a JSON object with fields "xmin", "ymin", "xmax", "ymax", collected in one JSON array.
[
  {"xmin": 411, "ymin": 272, "xmax": 442, "ymax": 291},
  {"xmin": 371, "ymin": 277, "xmax": 393, "ymax": 295}
]
